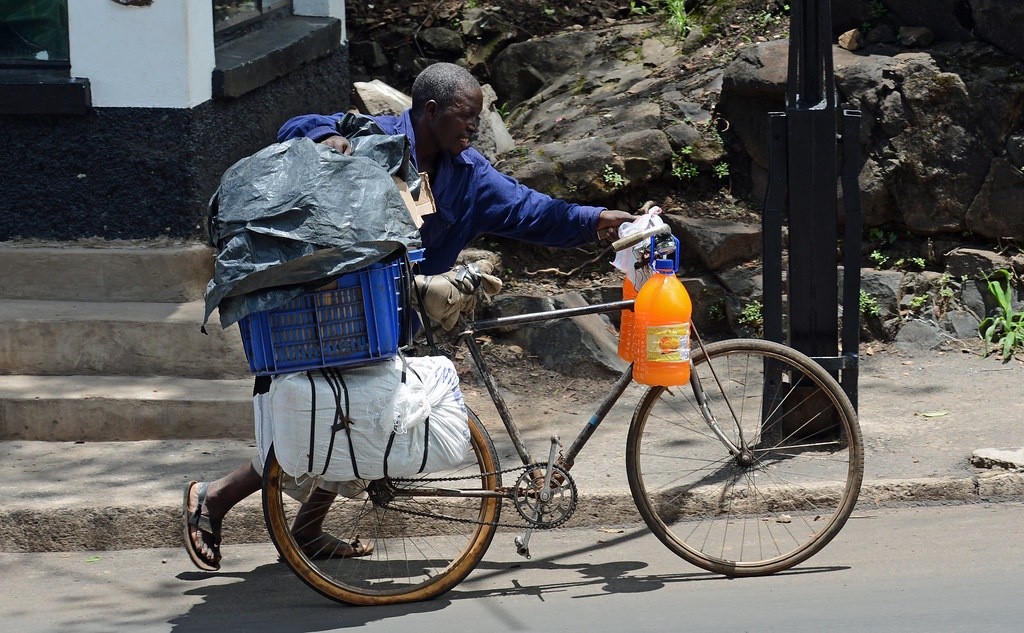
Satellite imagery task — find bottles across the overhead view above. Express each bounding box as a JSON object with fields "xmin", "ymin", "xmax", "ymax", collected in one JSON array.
[
  {"xmin": 618, "ymin": 276, "xmax": 638, "ymax": 361},
  {"xmin": 633, "ymin": 235, "xmax": 693, "ymax": 386}
]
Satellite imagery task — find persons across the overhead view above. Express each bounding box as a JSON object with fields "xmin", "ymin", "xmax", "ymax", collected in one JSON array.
[{"xmin": 182, "ymin": 63, "xmax": 638, "ymax": 571}]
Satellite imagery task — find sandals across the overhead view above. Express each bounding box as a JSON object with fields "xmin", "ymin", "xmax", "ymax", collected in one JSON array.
[
  {"xmin": 276, "ymin": 532, "xmax": 372, "ymax": 563},
  {"xmin": 182, "ymin": 481, "xmax": 222, "ymax": 571}
]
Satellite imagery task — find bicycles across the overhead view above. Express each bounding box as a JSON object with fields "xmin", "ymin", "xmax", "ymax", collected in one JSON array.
[{"xmin": 260, "ymin": 198, "xmax": 866, "ymax": 607}]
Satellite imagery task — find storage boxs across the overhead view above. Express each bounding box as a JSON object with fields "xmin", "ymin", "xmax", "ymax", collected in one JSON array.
[{"xmin": 238, "ymin": 261, "xmax": 404, "ymax": 377}]
